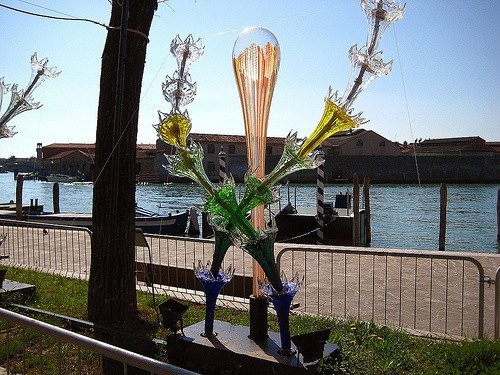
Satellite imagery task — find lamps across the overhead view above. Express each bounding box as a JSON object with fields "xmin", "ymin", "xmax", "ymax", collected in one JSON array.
[
  {"xmin": 152, "ymin": 0, "xmax": 408, "ymax": 375},
  {"xmin": 0, "ymin": 51, "xmax": 63, "ymax": 140}
]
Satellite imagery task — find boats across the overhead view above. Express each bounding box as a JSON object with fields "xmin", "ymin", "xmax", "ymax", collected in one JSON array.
[{"xmin": 0, "ymin": 180, "xmax": 190, "ymax": 237}]
